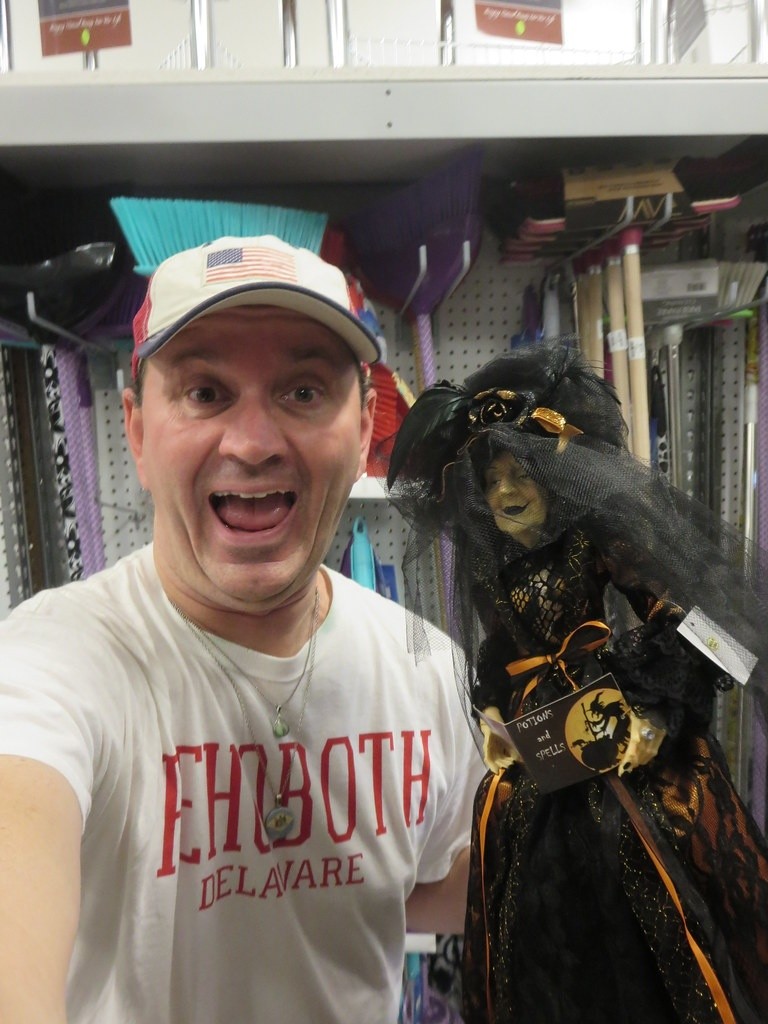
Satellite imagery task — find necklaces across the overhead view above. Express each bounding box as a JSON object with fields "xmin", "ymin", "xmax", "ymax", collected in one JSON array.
[{"xmin": 168, "ymin": 585, "xmax": 320, "ymax": 837}]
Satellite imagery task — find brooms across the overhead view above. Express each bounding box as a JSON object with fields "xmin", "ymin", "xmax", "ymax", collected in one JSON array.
[{"xmin": 1, "ymin": 182, "xmax": 768, "ymax": 1024}]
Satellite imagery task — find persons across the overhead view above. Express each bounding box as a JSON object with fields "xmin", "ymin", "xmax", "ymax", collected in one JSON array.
[
  {"xmin": 391, "ymin": 334, "xmax": 767, "ymax": 1023},
  {"xmin": 0, "ymin": 235, "xmax": 490, "ymax": 1023}
]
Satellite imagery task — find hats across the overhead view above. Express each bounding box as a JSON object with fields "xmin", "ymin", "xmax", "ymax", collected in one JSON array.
[{"xmin": 130, "ymin": 234, "xmax": 381, "ymax": 390}]
